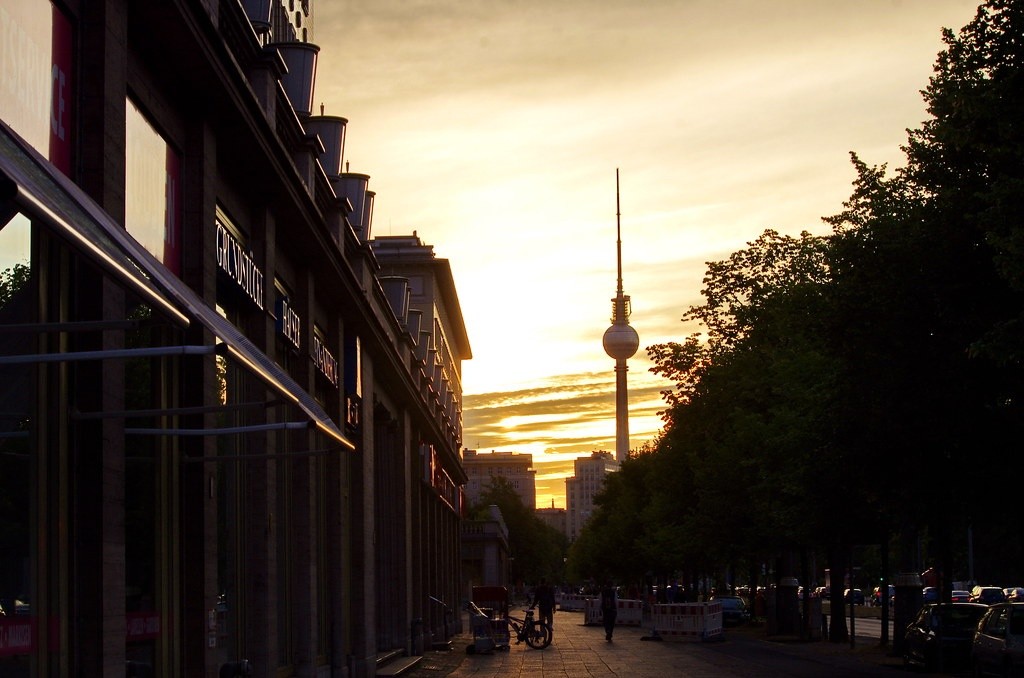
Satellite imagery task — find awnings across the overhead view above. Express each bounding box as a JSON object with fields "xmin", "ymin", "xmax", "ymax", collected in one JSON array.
[{"xmin": 0, "ymin": 121, "xmax": 356, "ymax": 457}]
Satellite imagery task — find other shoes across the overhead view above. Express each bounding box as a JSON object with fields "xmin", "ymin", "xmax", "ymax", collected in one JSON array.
[
  {"xmin": 606, "ymin": 631, "xmax": 613, "ymax": 641},
  {"xmin": 546, "ymin": 625, "xmax": 553, "ymax": 632}
]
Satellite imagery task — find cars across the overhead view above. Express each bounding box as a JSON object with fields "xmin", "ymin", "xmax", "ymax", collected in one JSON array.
[
  {"xmin": 1008, "ymin": 588, "xmax": 1024, "ymax": 603},
  {"xmin": 902, "ymin": 601, "xmax": 990, "ymax": 678},
  {"xmin": 952, "ymin": 590, "xmax": 972, "ymax": 603},
  {"xmin": 734, "ymin": 586, "xmax": 750, "ymax": 597},
  {"xmin": 1003, "ymin": 587, "xmax": 1016, "ymax": 597},
  {"xmin": 843, "ymin": 588, "xmax": 865, "ymax": 606},
  {"xmin": 923, "ymin": 586, "xmax": 937, "ymax": 604},
  {"xmin": 973, "ymin": 601, "xmax": 1024, "ymax": 678},
  {"xmin": 797, "ymin": 588, "xmax": 813, "ymax": 600},
  {"xmin": 813, "ymin": 586, "xmax": 828, "ymax": 599},
  {"xmin": 712, "ymin": 594, "xmax": 751, "ymax": 629}
]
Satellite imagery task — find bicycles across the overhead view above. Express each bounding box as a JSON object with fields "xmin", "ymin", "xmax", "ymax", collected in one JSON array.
[{"xmin": 508, "ymin": 609, "xmax": 554, "ymax": 649}]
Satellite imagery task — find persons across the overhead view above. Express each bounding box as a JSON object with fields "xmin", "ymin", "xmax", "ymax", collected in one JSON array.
[
  {"xmin": 600, "ymin": 580, "xmax": 618, "ymax": 641},
  {"xmin": 656, "ymin": 580, "xmax": 687, "ymax": 614},
  {"xmin": 530, "ymin": 577, "xmax": 556, "ymax": 632}
]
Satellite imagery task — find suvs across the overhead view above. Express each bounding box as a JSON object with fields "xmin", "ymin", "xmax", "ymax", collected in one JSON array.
[{"xmin": 965, "ymin": 584, "xmax": 1008, "ymax": 606}]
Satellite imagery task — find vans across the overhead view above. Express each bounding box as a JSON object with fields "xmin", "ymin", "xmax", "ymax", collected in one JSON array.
[{"xmin": 868, "ymin": 585, "xmax": 896, "ymax": 607}]
